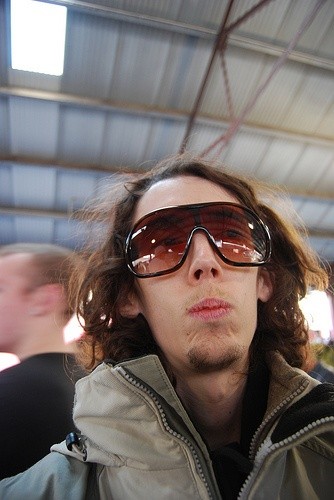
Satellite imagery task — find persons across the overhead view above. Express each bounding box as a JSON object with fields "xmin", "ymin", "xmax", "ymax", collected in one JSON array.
[
  {"xmin": 0, "ymin": 160, "xmax": 332, "ymax": 500},
  {"xmin": 2, "ymin": 242, "xmax": 88, "ymax": 480}
]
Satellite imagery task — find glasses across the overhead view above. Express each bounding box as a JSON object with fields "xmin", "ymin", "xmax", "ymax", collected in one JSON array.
[{"xmin": 122, "ymin": 200, "xmax": 272, "ymax": 278}]
